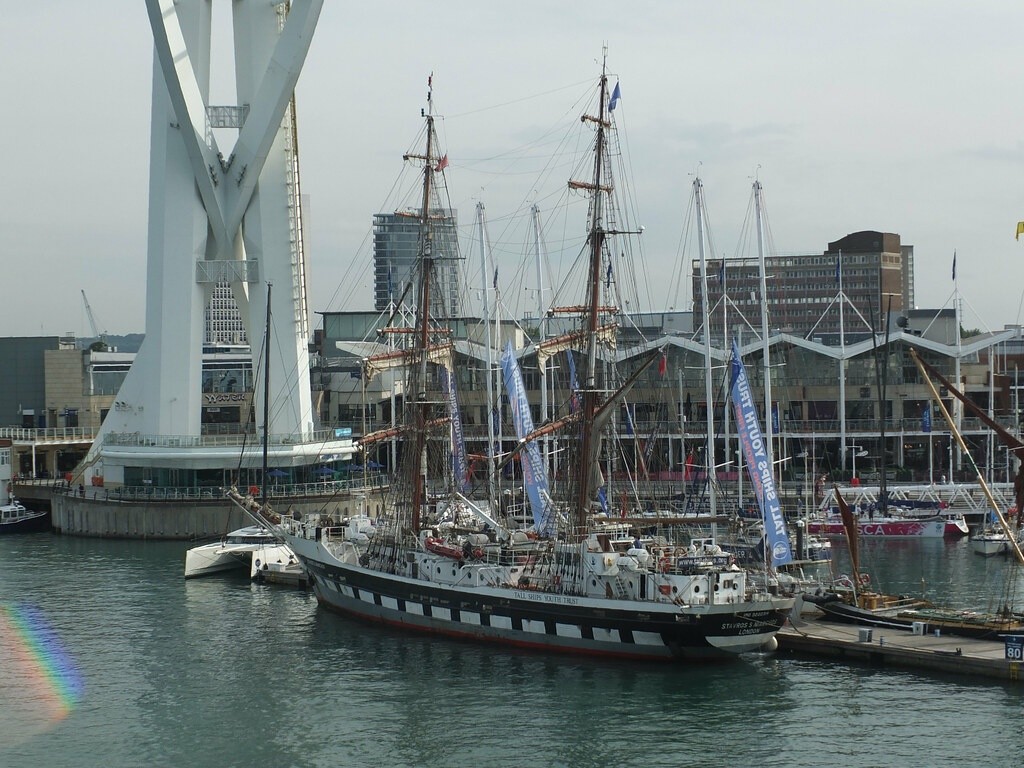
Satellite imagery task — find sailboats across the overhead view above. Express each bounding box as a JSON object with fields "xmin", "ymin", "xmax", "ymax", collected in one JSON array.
[{"xmin": 184, "ymin": 35, "xmax": 1024, "ymax": 666}]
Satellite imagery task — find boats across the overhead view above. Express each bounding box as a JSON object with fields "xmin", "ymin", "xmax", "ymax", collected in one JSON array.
[{"xmin": 0, "ymin": 485, "xmax": 50, "ymax": 532}]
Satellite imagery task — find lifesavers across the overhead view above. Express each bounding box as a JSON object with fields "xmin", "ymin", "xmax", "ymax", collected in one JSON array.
[
  {"xmin": 858, "ymin": 573, "xmax": 869, "ymax": 588},
  {"xmin": 658, "ymin": 558, "xmax": 670, "ymax": 573},
  {"xmin": 840, "ymin": 574, "xmax": 849, "ymax": 587}
]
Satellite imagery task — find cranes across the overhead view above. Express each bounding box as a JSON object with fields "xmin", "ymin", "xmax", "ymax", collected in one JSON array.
[{"xmin": 81, "ymin": 289, "xmax": 109, "ymax": 344}]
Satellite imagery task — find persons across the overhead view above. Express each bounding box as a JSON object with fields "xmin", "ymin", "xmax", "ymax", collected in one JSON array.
[
  {"xmin": 67, "ymin": 472, "xmax": 72, "ymax": 487},
  {"xmin": 869, "ymin": 504, "xmax": 874, "ymax": 523},
  {"xmin": 815, "ymin": 480, "xmax": 825, "ymax": 495},
  {"xmin": 798, "ymin": 499, "xmax": 803, "ymax": 515},
  {"xmin": 633, "ymin": 536, "xmax": 642, "ymax": 549},
  {"xmin": 78, "ymin": 484, "xmax": 84, "ymax": 497}
]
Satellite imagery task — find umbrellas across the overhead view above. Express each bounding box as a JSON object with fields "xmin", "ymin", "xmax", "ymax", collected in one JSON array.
[
  {"xmin": 337, "ymin": 463, "xmax": 365, "ymax": 480},
  {"xmin": 362, "ymin": 461, "xmax": 385, "ymax": 476},
  {"xmin": 266, "ymin": 469, "xmax": 290, "ymax": 490},
  {"xmin": 311, "ymin": 467, "xmax": 338, "ymax": 482}
]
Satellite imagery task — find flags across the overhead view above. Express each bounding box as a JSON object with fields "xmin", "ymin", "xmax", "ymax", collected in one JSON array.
[
  {"xmin": 922, "ymin": 401, "xmax": 932, "ymax": 432},
  {"xmin": 597, "ymin": 486, "xmax": 610, "ymax": 519},
  {"xmin": 1016, "ymin": 221, "xmax": 1024, "ymax": 242},
  {"xmin": 609, "ymin": 81, "xmax": 622, "ymax": 112},
  {"xmin": 607, "ymin": 264, "xmax": 611, "ymax": 288},
  {"xmin": 434, "ymin": 154, "xmax": 448, "ymax": 172},
  {"xmin": 771, "ymin": 405, "xmax": 781, "ymax": 433},
  {"xmin": 493, "ymin": 266, "xmax": 498, "ymax": 287}
]
